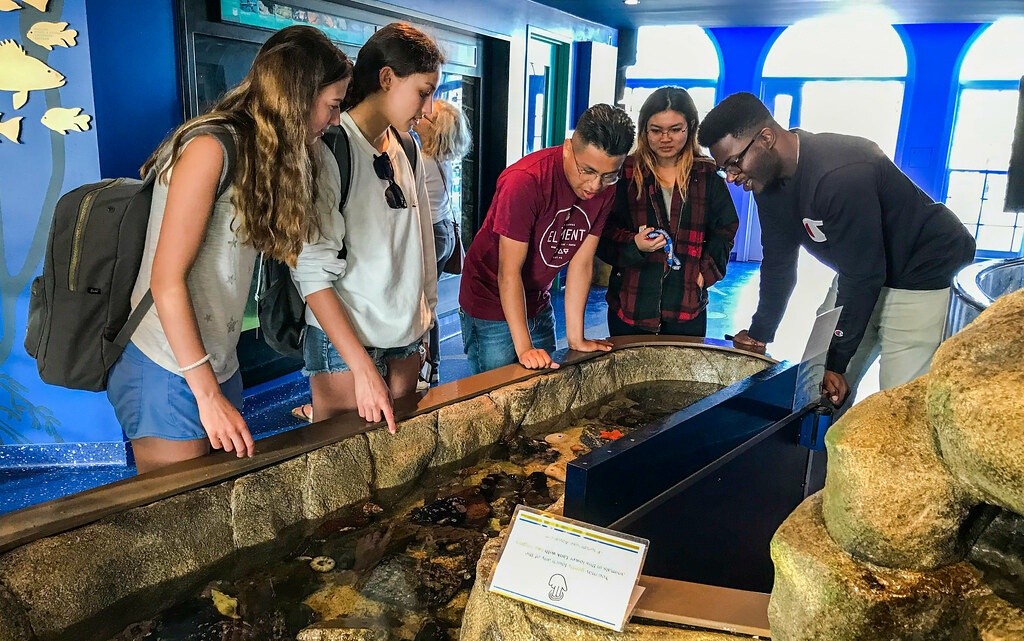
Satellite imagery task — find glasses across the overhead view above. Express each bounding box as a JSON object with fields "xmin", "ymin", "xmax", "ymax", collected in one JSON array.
[
  {"xmin": 570, "ymin": 138, "xmax": 623, "ymax": 186},
  {"xmin": 646, "ymin": 124, "xmax": 689, "ymax": 141},
  {"xmin": 715, "ymin": 129, "xmax": 763, "ymax": 179},
  {"xmin": 373, "ymin": 152, "xmax": 407, "ymax": 209}
]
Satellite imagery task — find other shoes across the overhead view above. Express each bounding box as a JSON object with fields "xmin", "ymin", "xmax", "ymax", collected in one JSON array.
[
  {"xmin": 431, "ymin": 374, "xmax": 439, "ymax": 383},
  {"xmin": 416, "ymin": 379, "xmax": 430, "ymax": 392}
]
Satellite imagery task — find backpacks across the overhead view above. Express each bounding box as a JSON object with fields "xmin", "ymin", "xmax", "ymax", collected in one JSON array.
[
  {"xmin": 258, "ymin": 124, "xmax": 417, "ymax": 361},
  {"xmin": 24, "ymin": 123, "xmax": 237, "ymax": 393}
]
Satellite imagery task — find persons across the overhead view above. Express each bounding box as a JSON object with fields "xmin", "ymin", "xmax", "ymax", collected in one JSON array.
[
  {"xmin": 106, "ymin": 25, "xmax": 352, "ymax": 476},
  {"xmin": 597, "ymin": 87, "xmax": 739, "ymax": 337},
  {"xmin": 458, "ymin": 103, "xmax": 635, "ymax": 375},
  {"xmin": 291, "ymin": 23, "xmax": 472, "ymax": 434},
  {"xmin": 696, "ymin": 91, "xmax": 976, "ymax": 426}
]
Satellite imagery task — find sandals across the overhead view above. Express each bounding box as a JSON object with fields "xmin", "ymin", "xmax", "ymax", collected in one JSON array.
[{"xmin": 292, "ymin": 405, "xmax": 312, "ymax": 423}]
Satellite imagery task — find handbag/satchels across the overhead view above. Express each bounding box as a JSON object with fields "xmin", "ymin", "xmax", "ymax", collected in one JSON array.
[{"xmin": 443, "ymin": 222, "xmax": 466, "ymax": 275}]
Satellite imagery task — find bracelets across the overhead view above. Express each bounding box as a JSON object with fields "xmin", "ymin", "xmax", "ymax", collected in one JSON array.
[{"xmin": 178, "ymin": 354, "xmax": 211, "ymax": 372}]
